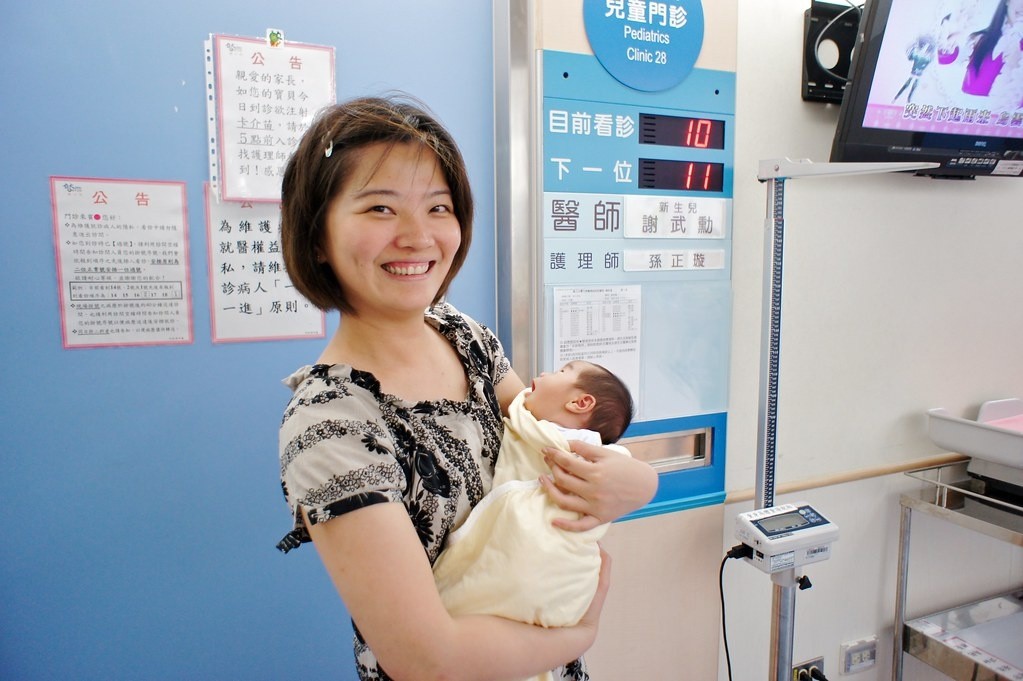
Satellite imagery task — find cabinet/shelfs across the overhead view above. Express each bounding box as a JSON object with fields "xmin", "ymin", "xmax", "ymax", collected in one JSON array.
[{"xmin": 891, "ymin": 461, "xmax": 1023, "ymax": 681}]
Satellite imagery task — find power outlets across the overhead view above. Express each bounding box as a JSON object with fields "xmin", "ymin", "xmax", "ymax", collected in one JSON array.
[
  {"xmin": 792, "ymin": 657, "xmax": 824, "ymax": 681},
  {"xmin": 838, "ymin": 635, "xmax": 877, "ymax": 675}
]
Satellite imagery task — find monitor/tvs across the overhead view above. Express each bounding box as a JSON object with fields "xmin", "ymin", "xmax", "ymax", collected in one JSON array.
[{"xmin": 829, "ymin": 0, "xmax": 1023, "ymax": 177}]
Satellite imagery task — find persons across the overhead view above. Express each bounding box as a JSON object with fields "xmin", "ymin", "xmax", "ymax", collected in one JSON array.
[
  {"xmin": 909, "ymin": 1, "xmax": 1023, "ymax": 114},
  {"xmin": 275, "ymin": 100, "xmax": 658, "ymax": 681},
  {"xmin": 432, "ymin": 359, "xmax": 634, "ymax": 629}
]
